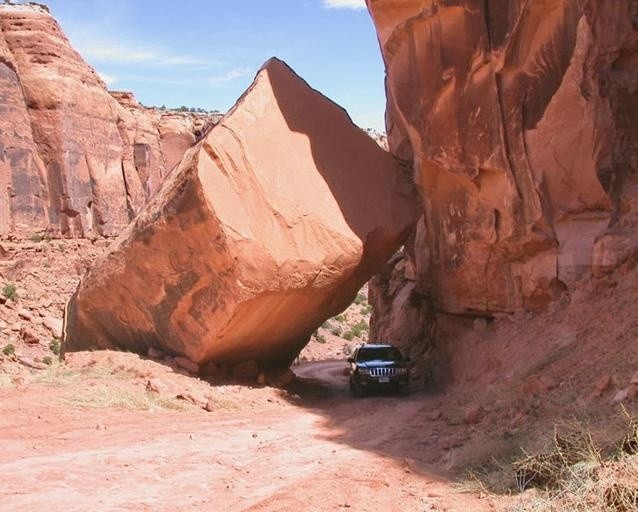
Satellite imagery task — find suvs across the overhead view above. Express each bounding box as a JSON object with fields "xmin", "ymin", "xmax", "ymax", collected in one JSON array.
[{"xmin": 346, "ymin": 343, "xmax": 408, "ymax": 396}]
